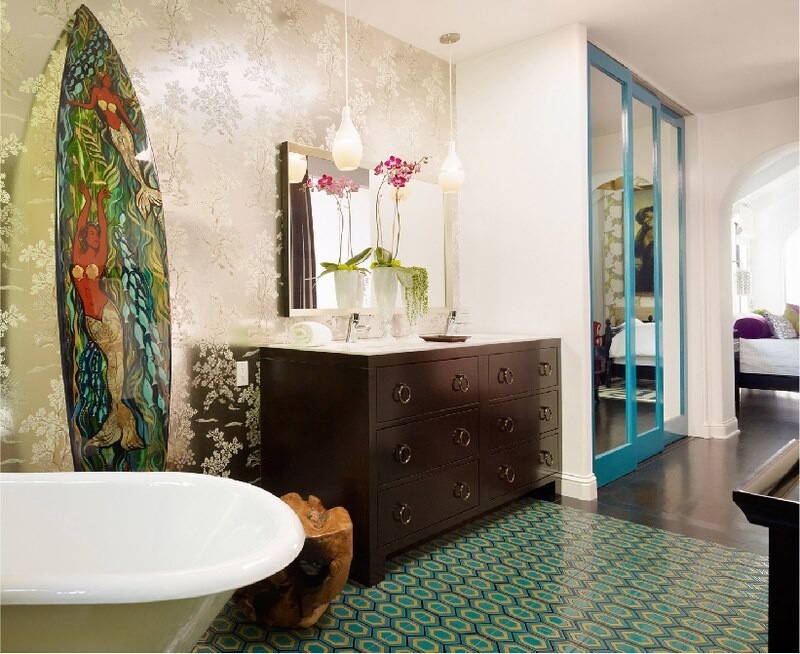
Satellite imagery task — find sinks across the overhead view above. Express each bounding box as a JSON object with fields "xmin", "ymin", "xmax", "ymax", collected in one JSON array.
[{"xmin": 419, "ymin": 335, "xmax": 472, "ymax": 343}]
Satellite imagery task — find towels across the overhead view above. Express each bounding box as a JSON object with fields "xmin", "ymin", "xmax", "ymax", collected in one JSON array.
[{"xmin": 290, "ymin": 321, "xmax": 333, "ymax": 346}]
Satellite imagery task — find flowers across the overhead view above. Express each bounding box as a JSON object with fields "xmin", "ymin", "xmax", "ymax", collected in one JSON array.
[
  {"xmin": 298, "ymin": 174, "xmax": 374, "ymax": 284},
  {"xmin": 369, "ymin": 156, "xmax": 429, "ymax": 324}
]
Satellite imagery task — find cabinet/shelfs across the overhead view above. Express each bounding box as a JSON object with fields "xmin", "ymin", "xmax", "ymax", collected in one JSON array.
[{"xmin": 259, "ymin": 339, "xmax": 562, "ymax": 587}]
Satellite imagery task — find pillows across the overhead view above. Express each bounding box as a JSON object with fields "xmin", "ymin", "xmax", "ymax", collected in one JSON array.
[{"xmin": 734, "ymin": 304, "xmax": 800, "ymax": 339}]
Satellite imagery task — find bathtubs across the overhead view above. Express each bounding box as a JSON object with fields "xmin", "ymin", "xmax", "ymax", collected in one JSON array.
[{"xmin": 3, "ymin": 466, "xmax": 307, "ymax": 654}]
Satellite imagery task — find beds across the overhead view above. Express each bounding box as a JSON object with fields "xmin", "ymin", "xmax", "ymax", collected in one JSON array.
[
  {"xmin": 733, "ymin": 337, "xmax": 800, "ymax": 418},
  {"xmin": 604, "ymin": 315, "xmax": 656, "ymax": 389}
]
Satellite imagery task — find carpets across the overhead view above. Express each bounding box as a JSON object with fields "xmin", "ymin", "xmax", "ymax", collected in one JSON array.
[{"xmin": 599, "ymin": 377, "xmax": 656, "ymax": 403}]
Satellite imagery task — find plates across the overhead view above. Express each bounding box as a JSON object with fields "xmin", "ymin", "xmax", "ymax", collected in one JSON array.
[{"xmin": 419, "ymin": 335, "xmax": 472, "ymax": 342}]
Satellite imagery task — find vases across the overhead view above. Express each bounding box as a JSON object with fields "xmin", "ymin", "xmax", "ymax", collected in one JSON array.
[
  {"xmin": 372, "ymin": 267, "xmax": 398, "ymax": 323},
  {"xmin": 334, "ymin": 271, "xmax": 363, "ymax": 308}
]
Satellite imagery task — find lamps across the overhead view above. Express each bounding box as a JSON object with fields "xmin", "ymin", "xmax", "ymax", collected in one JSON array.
[
  {"xmin": 332, "ymin": 0, "xmax": 362, "ymax": 171},
  {"xmin": 439, "ymin": 33, "xmax": 464, "ymax": 194}
]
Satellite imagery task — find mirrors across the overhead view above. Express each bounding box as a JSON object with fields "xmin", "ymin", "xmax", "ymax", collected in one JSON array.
[
  {"xmin": 282, "ymin": 141, "xmax": 453, "ymax": 318},
  {"xmin": 589, "ymin": 62, "xmax": 682, "ymax": 455}
]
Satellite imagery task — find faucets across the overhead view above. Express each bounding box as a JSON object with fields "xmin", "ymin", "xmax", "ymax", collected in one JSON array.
[
  {"xmin": 442, "ymin": 310, "xmax": 457, "ymax": 335},
  {"xmin": 345, "ymin": 312, "xmax": 372, "ymax": 343}
]
